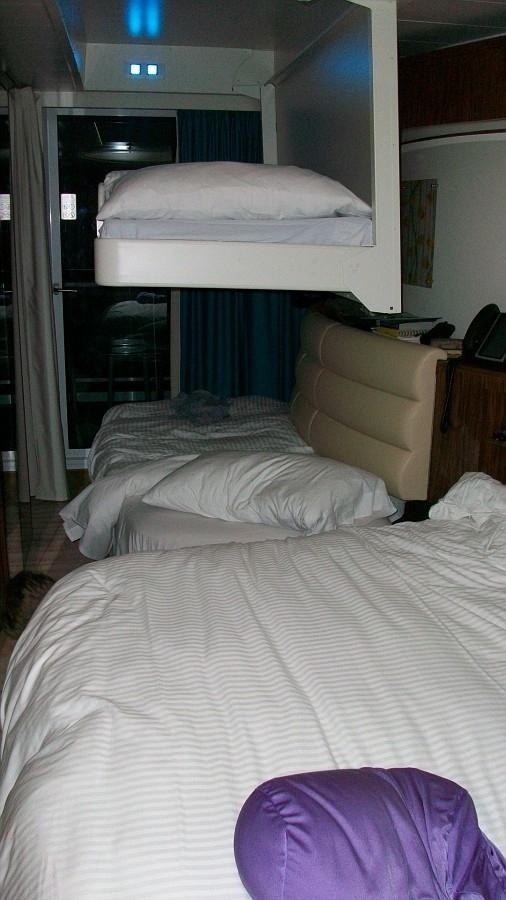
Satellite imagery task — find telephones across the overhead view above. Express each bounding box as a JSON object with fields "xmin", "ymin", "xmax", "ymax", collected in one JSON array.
[{"xmin": 462, "ymin": 304, "xmax": 506, "ymax": 372}]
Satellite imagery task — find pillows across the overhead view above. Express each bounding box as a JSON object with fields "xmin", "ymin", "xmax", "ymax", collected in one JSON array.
[
  {"xmin": 142, "ymin": 450, "xmax": 398, "ymax": 536},
  {"xmin": 96, "ymin": 160, "xmax": 373, "ymax": 229},
  {"xmin": 234, "ymin": 768, "xmax": 506, "ymax": 900}
]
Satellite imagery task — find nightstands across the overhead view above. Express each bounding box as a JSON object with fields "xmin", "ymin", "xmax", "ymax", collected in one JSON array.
[{"xmin": 426, "ymin": 358, "xmax": 506, "ymax": 505}]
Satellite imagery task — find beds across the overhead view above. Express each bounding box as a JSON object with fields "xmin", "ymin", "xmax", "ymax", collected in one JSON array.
[
  {"xmin": 90, "ymin": 158, "xmax": 373, "ymax": 294},
  {"xmin": 88, "ymin": 311, "xmax": 447, "ymax": 556},
  {"xmin": 1, "ymin": 511, "xmax": 506, "ymax": 900}
]
